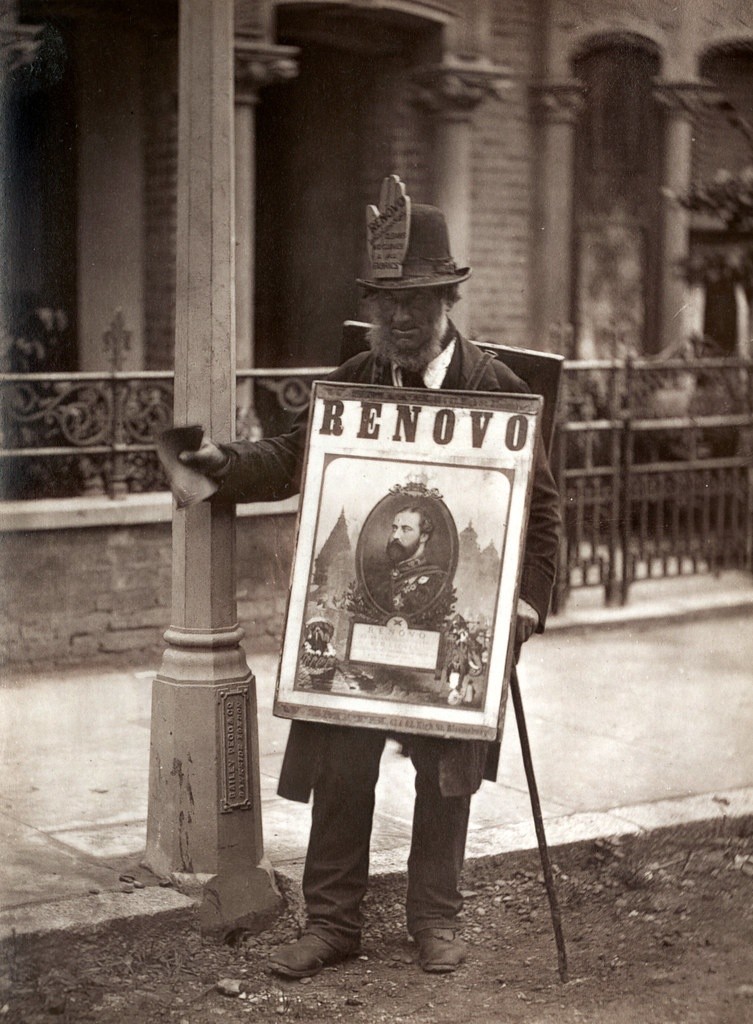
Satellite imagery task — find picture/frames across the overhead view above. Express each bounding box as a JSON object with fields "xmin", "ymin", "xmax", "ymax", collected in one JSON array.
[{"xmin": 272, "ymin": 380, "xmax": 545, "ymax": 740}]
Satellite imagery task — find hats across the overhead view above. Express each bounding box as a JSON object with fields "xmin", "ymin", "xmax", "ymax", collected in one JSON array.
[{"xmin": 355, "ymin": 175, "xmax": 472, "ymax": 290}]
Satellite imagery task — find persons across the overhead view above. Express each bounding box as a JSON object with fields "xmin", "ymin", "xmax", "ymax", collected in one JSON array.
[{"xmin": 177, "ymin": 177, "xmax": 563, "ymax": 981}]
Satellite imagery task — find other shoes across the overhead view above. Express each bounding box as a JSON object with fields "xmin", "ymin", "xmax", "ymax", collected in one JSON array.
[
  {"xmin": 269, "ymin": 933, "xmax": 340, "ymax": 977},
  {"xmin": 414, "ymin": 927, "xmax": 466, "ymax": 972}
]
